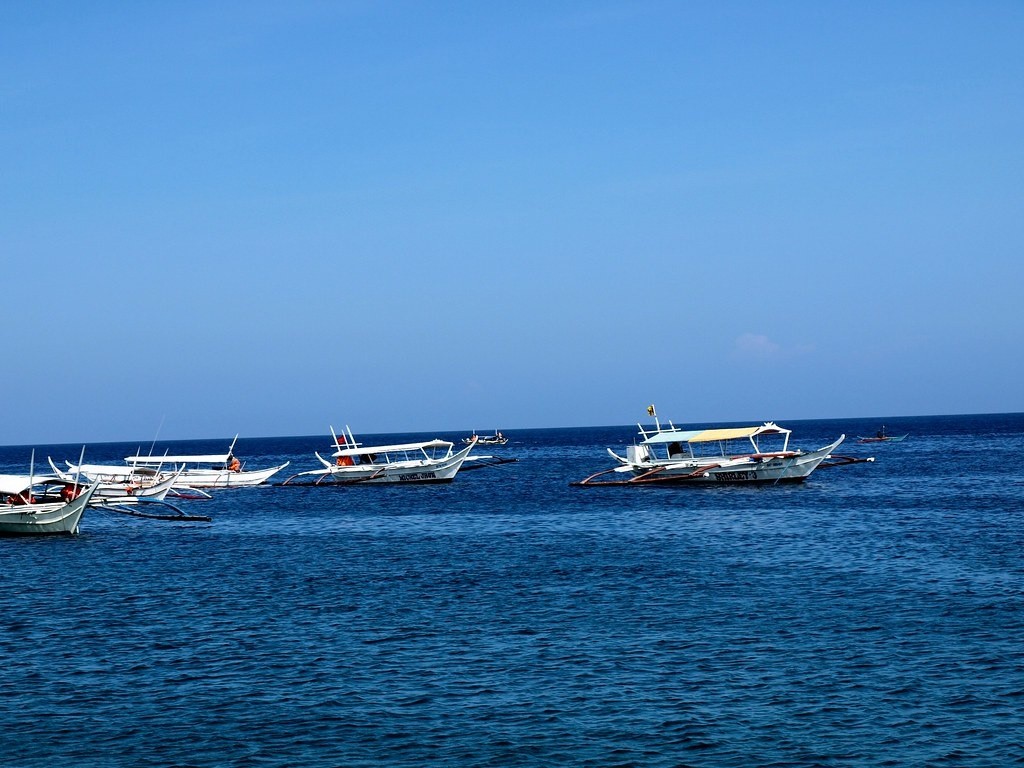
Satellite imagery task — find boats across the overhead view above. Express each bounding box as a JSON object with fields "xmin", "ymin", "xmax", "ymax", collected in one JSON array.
[
  {"xmin": 853, "ymin": 426, "xmax": 909, "ymax": 443},
  {"xmin": 280, "ymin": 425, "xmax": 478, "ymax": 483},
  {"xmin": 0, "ymin": 431, "xmax": 292, "ymax": 534},
  {"xmin": 462, "ymin": 428, "xmax": 509, "ymax": 445},
  {"xmin": 569, "ymin": 405, "xmax": 878, "ymax": 489}
]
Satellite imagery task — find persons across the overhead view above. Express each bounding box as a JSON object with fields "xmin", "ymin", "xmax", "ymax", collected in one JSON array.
[
  {"xmin": 668, "ymin": 442, "xmax": 687, "ymax": 458},
  {"xmin": 471, "ymin": 434, "xmax": 476, "ymax": 443},
  {"xmin": 5, "ymin": 488, "xmax": 36, "ymax": 507},
  {"xmin": 61, "ymin": 485, "xmax": 80, "ymax": 501},
  {"xmin": 498, "ymin": 432, "xmax": 506, "ymax": 443},
  {"xmin": 337, "ymin": 456, "xmax": 354, "ymax": 465},
  {"xmin": 125, "ymin": 487, "xmax": 133, "ymax": 495},
  {"xmin": 337, "ymin": 436, "xmax": 345, "ymax": 443},
  {"xmin": 228, "ymin": 455, "xmax": 240, "ymax": 471}
]
